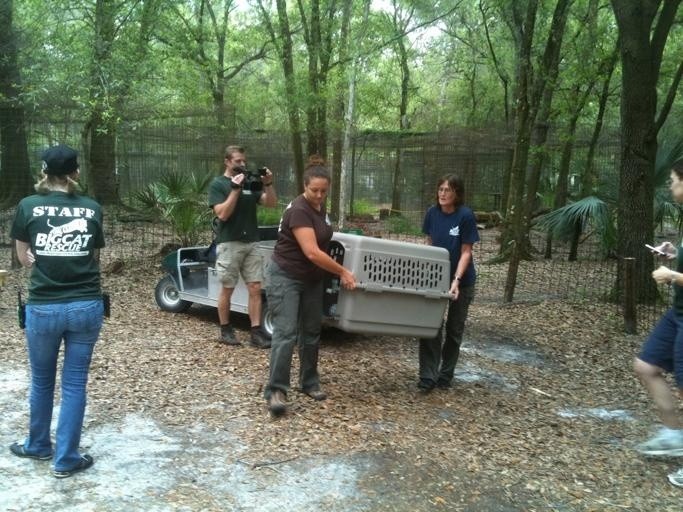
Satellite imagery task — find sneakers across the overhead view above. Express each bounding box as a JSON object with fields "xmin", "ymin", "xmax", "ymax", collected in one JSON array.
[
  {"xmin": 9, "ymin": 441, "xmax": 52, "ymax": 461},
  {"xmin": 53, "ymin": 455, "xmax": 94, "ymax": 479},
  {"xmin": 307, "ymin": 390, "xmax": 327, "ymax": 400},
  {"xmin": 268, "ymin": 390, "xmax": 286, "ymax": 414},
  {"xmin": 635, "ymin": 433, "xmax": 683, "ymax": 457},
  {"xmin": 438, "ymin": 378, "xmax": 451, "ymax": 389},
  {"xmin": 667, "ymin": 468, "xmax": 683, "ymax": 488},
  {"xmin": 418, "ymin": 377, "xmax": 435, "ymax": 392}
]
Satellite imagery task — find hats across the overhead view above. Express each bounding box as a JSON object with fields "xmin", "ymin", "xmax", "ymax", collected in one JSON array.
[{"xmin": 40, "ymin": 145, "xmax": 78, "ymax": 175}]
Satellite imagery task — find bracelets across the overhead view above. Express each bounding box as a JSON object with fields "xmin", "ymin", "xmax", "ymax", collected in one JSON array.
[
  {"xmin": 261, "ymin": 182, "xmax": 274, "ymax": 187},
  {"xmin": 670, "ymin": 270, "xmax": 678, "ymax": 287},
  {"xmin": 451, "ymin": 275, "xmax": 463, "ymax": 282}
]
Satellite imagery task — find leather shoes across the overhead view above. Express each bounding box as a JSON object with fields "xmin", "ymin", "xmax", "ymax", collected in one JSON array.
[
  {"xmin": 250, "ymin": 335, "xmax": 271, "ymax": 349},
  {"xmin": 219, "ymin": 334, "xmax": 240, "ymax": 345}
]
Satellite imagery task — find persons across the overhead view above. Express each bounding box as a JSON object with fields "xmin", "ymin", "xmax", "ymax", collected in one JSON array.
[
  {"xmin": 6, "ymin": 142, "xmax": 108, "ymax": 481},
  {"xmin": 418, "ymin": 173, "xmax": 478, "ymax": 392},
  {"xmin": 206, "ymin": 144, "xmax": 280, "ymax": 349},
  {"xmin": 631, "ymin": 155, "xmax": 683, "ymax": 490},
  {"xmin": 261, "ymin": 159, "xmax": 359, "ymax": 418}
]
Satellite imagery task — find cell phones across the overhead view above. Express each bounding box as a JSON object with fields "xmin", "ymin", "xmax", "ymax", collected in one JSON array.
[{"xmin": 644, "ymin": 243, "xmax": 667, "ymax": 256}]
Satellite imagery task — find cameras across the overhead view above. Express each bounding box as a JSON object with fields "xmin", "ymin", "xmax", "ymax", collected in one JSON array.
[{"xmin": 232, "ymin": 166, "xmax": 267, "ymax": 193}]
